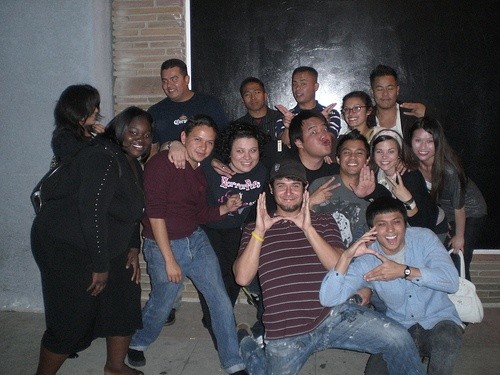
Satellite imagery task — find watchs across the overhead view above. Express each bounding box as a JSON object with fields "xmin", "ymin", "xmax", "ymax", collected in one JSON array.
[
  {"xmin": 404, "ymin": 199, "xmax": 417, "ymax": 210},
  {"xmin": 403, "ymin": 265, "xmax": 411, "ymax": 279}
]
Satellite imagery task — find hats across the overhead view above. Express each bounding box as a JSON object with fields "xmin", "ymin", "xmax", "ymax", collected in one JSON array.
[{"xmin": 270, "ymin": 160, "xmax": 307, "ymax": 185}]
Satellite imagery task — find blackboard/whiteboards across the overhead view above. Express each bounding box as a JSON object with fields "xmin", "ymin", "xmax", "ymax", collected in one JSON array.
[{"xmin": 190, "ymin": 1, "xmax": 500, "ymax": 251}]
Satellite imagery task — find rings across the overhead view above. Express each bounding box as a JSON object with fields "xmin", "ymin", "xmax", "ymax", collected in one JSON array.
[{"xmin": 222, "ymin": 166, "xmax": 225, "ymax": 168}]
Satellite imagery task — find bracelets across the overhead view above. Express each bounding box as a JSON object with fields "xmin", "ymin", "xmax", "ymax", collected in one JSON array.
[
  {"xmin": 166, "ymin": 139, "xmax": 175, "ymax": 150},
  {"xmin": 252, "ymin": 230, "xmax": 266, "ymax": 242}
]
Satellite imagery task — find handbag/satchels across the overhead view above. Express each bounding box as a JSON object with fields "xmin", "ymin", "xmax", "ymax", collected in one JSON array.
[{"xmin": 446, "ymin": 247, "xmax": 484, "ymax": 324}]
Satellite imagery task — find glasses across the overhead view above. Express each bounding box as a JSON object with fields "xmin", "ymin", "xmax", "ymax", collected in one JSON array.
[{"xmin": 342, "ymin": 104, "xmax": 367, "ymax": 114}]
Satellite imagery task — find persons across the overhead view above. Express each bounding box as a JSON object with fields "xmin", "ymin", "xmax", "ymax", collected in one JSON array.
[
  {"xmin": 271, "ymin": 109, "xmax": 346, "ymax": 212},
  {"xmin": 30, "ymin": 106, "xmax": 154, "ymax": 375},
  {"xmin": 367, "ymin": 63, "xmax": 419, "ymax": 143},
  {"xmin": 333, "ymin": 91, "xmax": 404, "ymax": 145},
  {"xmin": 144, "ymin": 58, "xmax": 229, "ymax": 326},
  {"xmin": 49, "ymin": 84, "xmax": 106, "ymax": 169},
  {"xmin": 319, "ymin": 197, "xmax": 464, "ymax": 375},
  {"xmin": 127, "ymin": 115, "xmax": 244, "ymax": 375},
  {"xmin": 214, "ymin": 77, "xmax": 294, "ymax": 171},
  {"xmin": 274, "ymin": 66, "xmax": 344, "ymax": 150},
  {"xmin": 400, "ymin": 101, "xmax": 487, "ymax": 325},
  {"xmin": 160, "ymin": 130, "xmax": 279, "ymax": 351},
  {"xmin": 307, "ymin": 130, "xmax": 387, "ymax": 261},
  {"xmin": 365, "ymin": 128, "xmax": 441, "ymax": 233},
  {"xmin": 233, "ymin": 157, "xmax": 426, "ymax": 375}
]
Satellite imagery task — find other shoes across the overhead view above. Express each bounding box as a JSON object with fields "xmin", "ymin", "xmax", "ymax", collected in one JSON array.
[
  {"xmin": 237, "ymin": 322, "xmax": 252, "ymax": 348},
  {"xmin": 164, "ymin": 309, "xmax": 176, "ymax": 326},
  {"xmin": 230, "ymin": 368, "xmax": 250, "ymax": 375},
  {"xmin": 127, "ymin": 348, "xmax": 146, "ymax": 366}
]
